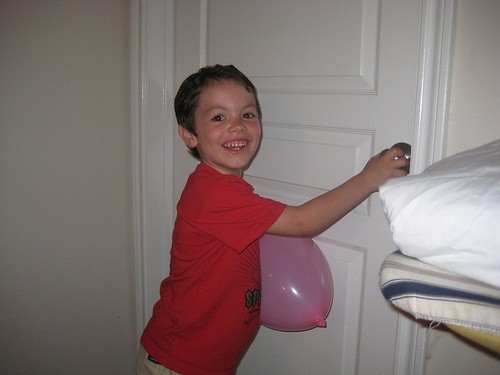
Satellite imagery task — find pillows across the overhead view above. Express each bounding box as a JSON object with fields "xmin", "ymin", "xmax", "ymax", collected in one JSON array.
[{"xmin": 376, "ymin": 135, "xmax": 500, "ymax": 293}]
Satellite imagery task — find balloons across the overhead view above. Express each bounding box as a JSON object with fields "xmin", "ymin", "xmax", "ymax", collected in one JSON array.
[{"xmin": 258, "ymin": 231, "xmax": 336, "ymax": 333}]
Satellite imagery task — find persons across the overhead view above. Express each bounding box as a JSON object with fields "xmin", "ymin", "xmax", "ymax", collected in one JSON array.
[{"xmin": 134, "ymin": 65, "xmax": 408, "ymax": 375}]
